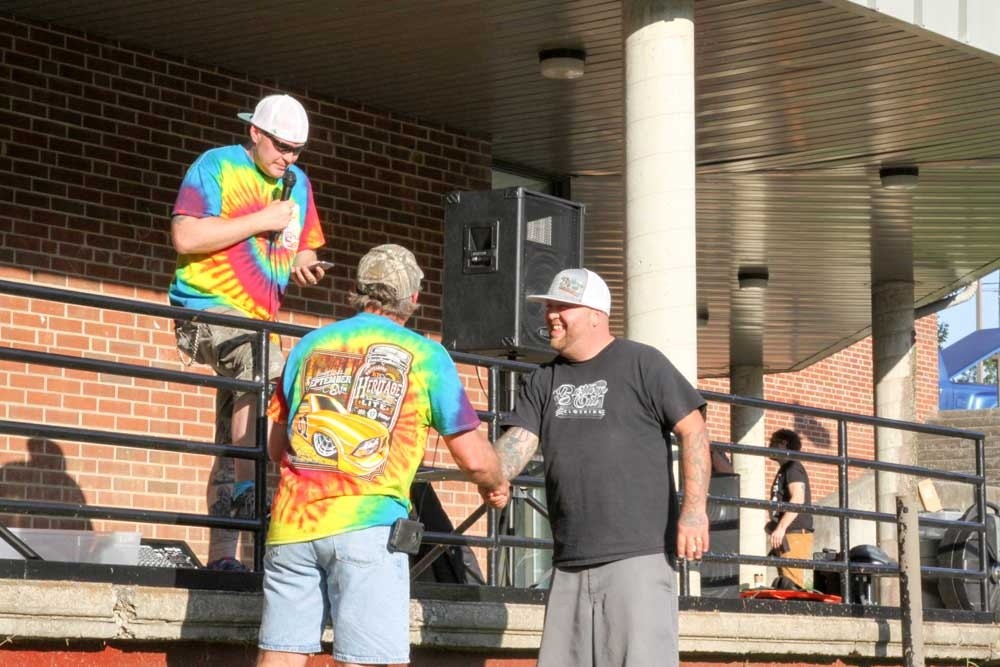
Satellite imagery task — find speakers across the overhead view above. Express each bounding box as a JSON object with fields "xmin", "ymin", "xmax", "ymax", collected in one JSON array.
[
  {"xmin": 441, "ymin": 186, "xmax": 586, "ymax": 367},
  {"xmin": 706, "ymin": 472, "xmax": 740, "ymax": 601},
  {"xmin": 812, "ymin": 552, "xmax": 879, "ymax": 607}
]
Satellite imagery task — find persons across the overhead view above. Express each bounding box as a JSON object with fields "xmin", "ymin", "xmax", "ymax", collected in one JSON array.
[
  {"xmin": 478, "ymin": 268, "xmax": 711, "ymax": 667},
  {"xmin": 167, "ymin": 95, "xmax": 325, "ymax": 573},
  {"xmin": 767, "ymin": 429, "xmax": 815, "ymax": 588},
  {"xmin": 256, "ymin": 243, "xmax": 510, "ymax": 667}
]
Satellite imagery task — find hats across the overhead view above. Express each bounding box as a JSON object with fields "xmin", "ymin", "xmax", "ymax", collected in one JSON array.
[
  {"xmin": 528, "ymin": 268, "xmax": 611, "ymax": 317},
  {"xmin": 357, "ymin": 243, "xmax": 424, "ymax": 300},
  {"xmin": 236, "ymin": 93, "xmax": 309, "ymax": 143}
]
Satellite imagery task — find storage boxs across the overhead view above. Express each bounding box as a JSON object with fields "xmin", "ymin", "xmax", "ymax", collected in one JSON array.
[{"xmin": 0, "ymin": 527, "xmax": 142, "ymax": 566}]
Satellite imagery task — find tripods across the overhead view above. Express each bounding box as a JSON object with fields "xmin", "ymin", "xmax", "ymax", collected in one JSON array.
[{"xmin": 407, "ymin": 366, "xmax": 548, "ymax": 585}]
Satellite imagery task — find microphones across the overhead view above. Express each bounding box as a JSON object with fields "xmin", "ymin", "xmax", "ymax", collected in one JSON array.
[{"xmin": 270, "ymin": 171, "xmax": 295, "ymax": 241}]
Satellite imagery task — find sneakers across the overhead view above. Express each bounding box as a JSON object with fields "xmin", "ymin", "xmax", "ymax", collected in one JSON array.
[
  {"xmin": 207, "ymin": 557, "xmax": 250, "ymax": 572},
  {"xmin": 228, "ymin": 485, "xmax": 272, "ymax": 520}
]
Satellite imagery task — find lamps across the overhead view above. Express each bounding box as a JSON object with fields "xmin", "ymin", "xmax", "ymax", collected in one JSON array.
[
  {"xmin": 697, "ymin": 311, "xmax": 710, "ymax": 329},
  {"xmin": 877, "ymin": 164, "xmax": 919, "ymax": 190},
  {"xmin": 538, "ymin": 47, "xmax": 588, "ymax": 80},
  {"xmin": 737, "ymin": 268, "xmax": 768, "ymax": 290}
]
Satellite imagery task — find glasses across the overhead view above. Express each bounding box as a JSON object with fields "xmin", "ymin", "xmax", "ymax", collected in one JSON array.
[{"xmin": 261, "ymin": 130, "xmax": 306, "ymax": 155}]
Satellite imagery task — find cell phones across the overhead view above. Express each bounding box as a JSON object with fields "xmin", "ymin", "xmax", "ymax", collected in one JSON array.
[
  {"xmin": 291, "ymin": 261, "xmax": 334, "ymax": 280},
  {"xmin": 390, "ymin": 518, "xmax": 424, "ymax": 555}
]
